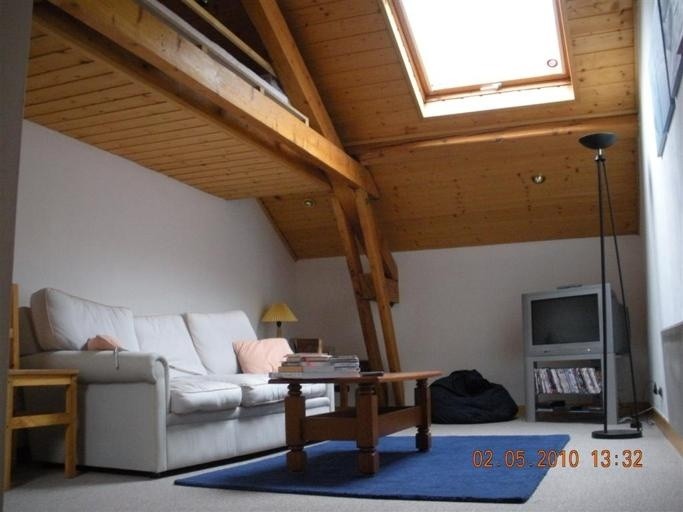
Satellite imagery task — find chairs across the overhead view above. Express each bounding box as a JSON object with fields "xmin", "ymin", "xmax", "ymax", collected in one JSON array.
[{"xmin": 0, "ymin": 283, "xmax": 80, "ymax": 492}]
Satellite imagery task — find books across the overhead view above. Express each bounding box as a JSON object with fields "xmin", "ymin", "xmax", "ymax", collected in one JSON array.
[
  {"xmin": 265, "ymin": 350, "xmax": 362, "ymax": 383},
  {"xmin": 533, "ymin": 365, "xmax": 604, "ymax": 414}
]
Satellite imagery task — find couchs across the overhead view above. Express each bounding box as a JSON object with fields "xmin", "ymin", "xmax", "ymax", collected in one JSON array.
[{"xmin": 10, "ymin": 306, "xmax": 337, "ymax": 479}]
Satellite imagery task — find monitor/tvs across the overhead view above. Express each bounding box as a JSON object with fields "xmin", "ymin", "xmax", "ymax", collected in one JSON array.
[{"xmin": 521, "ymin": 283, "xmax": 632, "ymax": 358}]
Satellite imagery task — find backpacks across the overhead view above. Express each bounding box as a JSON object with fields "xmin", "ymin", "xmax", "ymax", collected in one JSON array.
[{"xmin": 428, "ymin": 368, "xmax": 521, "ymax": 425}]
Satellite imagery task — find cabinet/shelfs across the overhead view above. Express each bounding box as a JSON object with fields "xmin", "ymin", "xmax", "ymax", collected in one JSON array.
[{"xmin": 525, "ymin": 353, "xmax": 628, "ymax": 425}]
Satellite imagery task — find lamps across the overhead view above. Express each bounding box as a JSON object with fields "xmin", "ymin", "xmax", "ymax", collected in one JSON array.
[
  {"xmin": 261, "ymin": 303, "xmax": 299, "ymax": 338},
  {"xmin": 577, "ymin": 131, "xmax": 645, "ymax": 439}
]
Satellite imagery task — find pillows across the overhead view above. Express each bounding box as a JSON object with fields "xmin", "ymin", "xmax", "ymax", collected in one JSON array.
[{"xmin": 30, "ymin": 286, "xmax": 295, "ymax": 382}]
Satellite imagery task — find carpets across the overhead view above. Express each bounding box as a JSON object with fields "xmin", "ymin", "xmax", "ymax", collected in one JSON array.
[{"xmin": 171, "ymin": 433, "xmax": 571, "ymax": 504}]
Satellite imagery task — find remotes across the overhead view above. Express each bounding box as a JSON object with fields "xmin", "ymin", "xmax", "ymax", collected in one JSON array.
[{"xmin": 360, "ymin": 371, "xmax": 385, "ymax": 377}]
